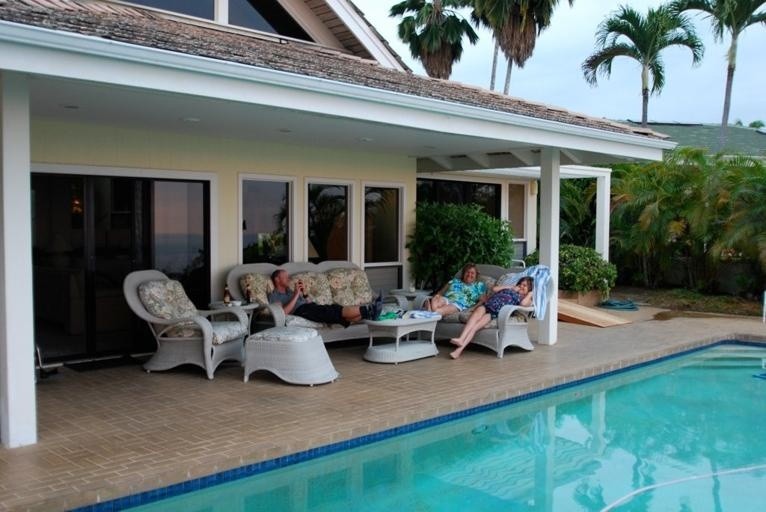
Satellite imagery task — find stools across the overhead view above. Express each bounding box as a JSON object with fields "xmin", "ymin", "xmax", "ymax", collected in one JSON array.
[{"xmin": 243, "ymin": 327, "xmax": 338, "ymax": 386}]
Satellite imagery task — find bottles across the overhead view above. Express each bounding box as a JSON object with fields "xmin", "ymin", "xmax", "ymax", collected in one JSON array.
[
  {"xmin": 246, "ymin": 284, "xmax": 253, "ymax": 303},
  {"xmin": 223, "ymin": 283, "xmax": 231, "ymax": 304}
]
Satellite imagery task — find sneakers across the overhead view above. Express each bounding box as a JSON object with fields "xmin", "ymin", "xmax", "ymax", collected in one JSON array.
[{"xmin": 371, "ymin": 290, "xmax": 383, "ymax": 320}]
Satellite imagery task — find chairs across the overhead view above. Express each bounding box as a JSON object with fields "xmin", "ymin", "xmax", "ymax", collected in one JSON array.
[{"xmin": 123, "ymin": 270, "xmax": 249, "ymax": 379}]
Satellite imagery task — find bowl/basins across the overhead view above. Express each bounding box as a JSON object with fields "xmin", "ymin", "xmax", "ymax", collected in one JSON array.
[{"xmin": 231, "ymin": 300, "xmax": 242, "ymax": 306}]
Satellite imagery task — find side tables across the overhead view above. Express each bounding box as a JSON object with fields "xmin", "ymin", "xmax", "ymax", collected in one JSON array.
[
  {"xmin": 209, "ymin": 301, "xmax": 260, "ymax": 334},
  {"xmin": 389, "ymin": 289, "xmax": 434, "ymax": 299}
]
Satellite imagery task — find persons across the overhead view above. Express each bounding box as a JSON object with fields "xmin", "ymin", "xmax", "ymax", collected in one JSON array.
[
  {"xmin": 268, "ymin": 268, "xmax": 385, "ymax": 329},
  {"xmin": 430, "ymin": 265, "xmax": 486, "ymax": 317},
  {"xmin": 449, "ymin": 275, "xmax": 534, "ymax": 358}
]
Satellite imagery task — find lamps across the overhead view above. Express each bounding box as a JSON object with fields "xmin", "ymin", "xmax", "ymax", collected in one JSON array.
[{"xmin": 530, "ymin": 178, "xmax": 538, "ymax": 195}]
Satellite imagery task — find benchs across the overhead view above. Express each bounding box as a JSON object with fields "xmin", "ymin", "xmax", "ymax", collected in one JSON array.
[
  {"xmin": 413, "ymin": 264, "xmax": 551, "ymax": 359},
  {"xmin": 226, "ymin": 261, "xmax": 408, "ymax": 344}
]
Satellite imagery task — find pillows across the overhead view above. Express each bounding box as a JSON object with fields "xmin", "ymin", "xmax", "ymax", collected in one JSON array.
[
  {"xmin": 139, "ymin": 280, "xmax": 197, "ymax": 333},
  {"xmin": 240, "ymin": 268, "xmax": 373, "ymax": 315}
]
